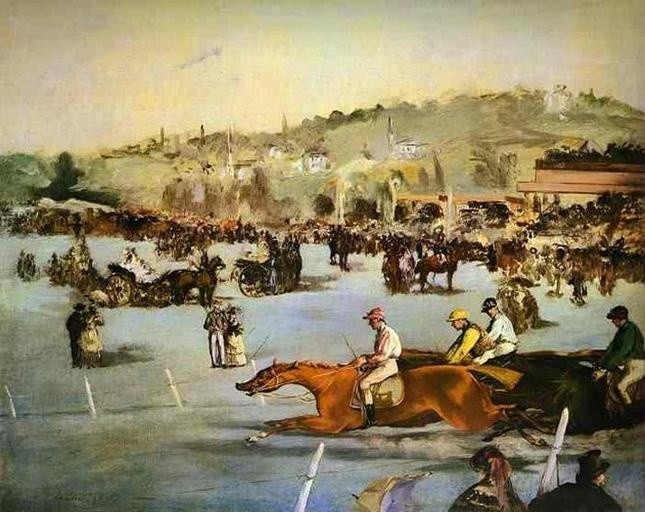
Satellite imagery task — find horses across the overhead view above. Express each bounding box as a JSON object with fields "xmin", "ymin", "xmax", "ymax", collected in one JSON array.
[
  {"xmin": 382, "ymin": 245, "xmax": 468, "ymax": 295},
  {"xmin": 166, "ymin": 255, "xmax": 225, "ymax": 309},
  {"xmin": 330, "ymin": 233, "xmax": 351, "ymax": 272}
]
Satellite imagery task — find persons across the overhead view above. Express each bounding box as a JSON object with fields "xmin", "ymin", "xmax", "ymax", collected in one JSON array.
[
  {"xmin": 593, "ymin": 302, "xmax": 645, "ymax": 405},
  {"xmin": 522, "ymin": 449, "xmax": 623, "ymax": 511},
  {"xmin": 354, "ymin": 305, "xmax": 401, "ymax": 428},
  {"xmin": 0, "ymin": 203, "xmax": 645, "ymax": 370},
  {"xmin": 444, "ymin": 444, "xmax": 525, "ymax": 510}
]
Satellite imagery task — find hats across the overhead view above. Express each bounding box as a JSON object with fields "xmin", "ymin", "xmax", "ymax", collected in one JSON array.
[
  {"xmin": 481, "ymin": 298, "xmax": 496, "ymax": 312},
  {"xmin": 447, "ymin": 309, "xmax": 468, "ymax": 322},
  {"xmin": 74, "ymin": 303, "xmax": 85, "ymax": 310},
  {"xmin": 575, "ymin": 449, "xmax": 609, "ymax": 481},
  {"xmin": 362, "ymin": 307, "xmax": 384, "ymax": 321}
]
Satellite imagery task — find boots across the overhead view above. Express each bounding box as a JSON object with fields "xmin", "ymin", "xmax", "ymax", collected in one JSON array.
[
  {"xmin": 620, "ymin": 405, "xmax": 632, "ymax": 424},
  {"xmin": 361, "ymin": 405, "xmax": 376, "ymax": 430}
]
